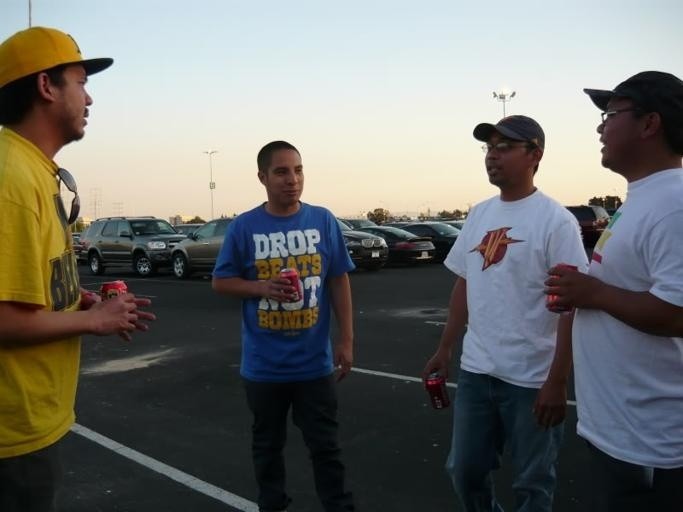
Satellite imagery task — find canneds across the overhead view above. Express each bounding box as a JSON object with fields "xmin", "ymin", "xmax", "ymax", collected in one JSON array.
[
  {"xmin": 424, "ymin": 372, "xmax": 451, "ymax": 410},
  {"xmin": 99, "ymin": 279, "xmax": 129, "ymax": 301},
  {"xmin": 545, "ymin": 264, "xmax": 579, "ymax": 313},
  {"xmin": 278, "ymin": 269, "xmax": 303, "ymax": 304}
]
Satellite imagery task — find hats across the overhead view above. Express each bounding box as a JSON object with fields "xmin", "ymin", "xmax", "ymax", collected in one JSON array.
[
  {"xmin": 583, "ymin": 71, "xmax": 682, "ymax": 110},
  {"xmin": 0, "ymin": 26, "xmax": 114, "ymax": 87},
  {"xmin": 473, "ymin": 115, "xmax": 544, "ymax": 151}
]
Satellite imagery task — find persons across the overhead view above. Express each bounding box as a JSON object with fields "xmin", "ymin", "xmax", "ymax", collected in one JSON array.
[
  {"xmin": 0, "ymin": 25, "xmax": 157, "ymax": 512},
  {"xmin": 423, "ymin": 114, "xmax": 590, "ymax": 512},
  {"xmin": 543, "ymin": 71, "xmax": 683, "ymax": 512},
  {"xmin": 211, "ymin": 141, "xmax": 356, "ymax": 512}
]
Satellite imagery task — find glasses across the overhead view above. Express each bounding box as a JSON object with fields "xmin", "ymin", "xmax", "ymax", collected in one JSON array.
[
  {"xmin": 56, "ymin": 169, "xmax": 81, "ymax": 227},
  {"xmin": 600, "ymin": 107, "xmax": 630, "ymax": 123},
  {"xmin": 480, "ymin": 141, "xmax": 525, "ymax": 154}
]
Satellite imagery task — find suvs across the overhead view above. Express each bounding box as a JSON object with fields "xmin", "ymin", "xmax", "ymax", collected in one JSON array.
[
  {"xmin": 327, "ymin": 219, "xmax": 389, "ymax": 272},
  {"xmin": 84, "ymin": 216, "xmax": 188, "ymax": 276},
  {"xmin": 564, "ymin": 206, "xmax": 610, "ymax": 240},
  {"xmin": 171, "ymin": 219, "xmax": 237, "ymax": 279}
]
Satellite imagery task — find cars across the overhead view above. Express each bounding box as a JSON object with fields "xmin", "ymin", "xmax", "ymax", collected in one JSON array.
[
  {"xmin": 72, "ymin": 237, "xmax": 85, "ymax": 261},
  {"xmin": 357, "ymin": 225, "xmax": 435, "ymax": 267},
  {"xmin": 402, "ymin": 222, "xmax": 461, "ymax": 250}
]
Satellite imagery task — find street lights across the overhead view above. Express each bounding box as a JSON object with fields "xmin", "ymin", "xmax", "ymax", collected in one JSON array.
[
  {"xmin": 204, "ymin": 150, "xmax": 220, "ymax": 221},
  {"xmin": 492, "ymin": 90, "xmax": 515, "ymax": 119}
]
